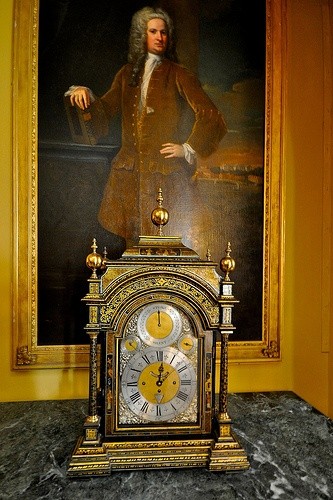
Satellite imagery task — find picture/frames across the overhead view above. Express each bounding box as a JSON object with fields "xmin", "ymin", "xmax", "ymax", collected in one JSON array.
[{"xmin": 12, "ymin": 0, "xmax": 287, "ymax": 371}]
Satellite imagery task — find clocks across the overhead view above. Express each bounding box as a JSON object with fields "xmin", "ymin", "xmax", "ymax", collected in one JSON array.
[{"xmin": 67, "ymin": 186, "xmax": 250, "ymax": 477}]
